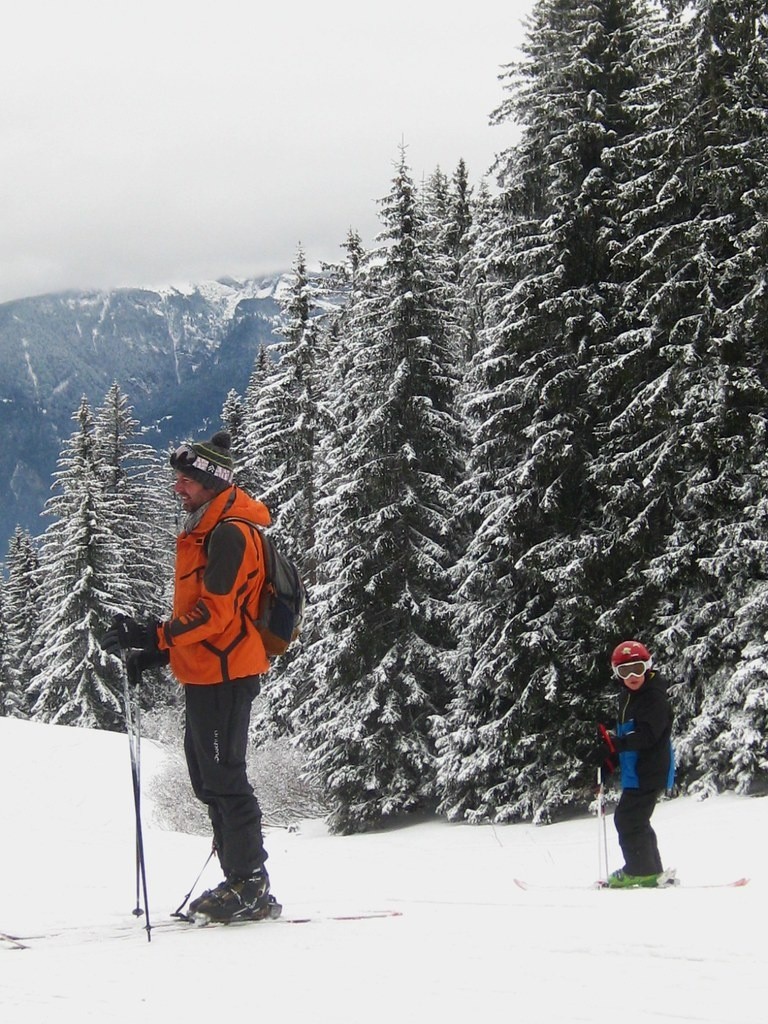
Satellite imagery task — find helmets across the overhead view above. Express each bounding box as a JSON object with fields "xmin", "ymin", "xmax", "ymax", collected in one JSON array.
[{"xmin": 611, "ymin": 641, "xmax": 652, "ymax": 673}]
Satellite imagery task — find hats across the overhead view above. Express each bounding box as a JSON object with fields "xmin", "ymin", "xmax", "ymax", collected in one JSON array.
[{"xmin": 170, "ymin": 431, "xmax": 234, "ymax": 490}]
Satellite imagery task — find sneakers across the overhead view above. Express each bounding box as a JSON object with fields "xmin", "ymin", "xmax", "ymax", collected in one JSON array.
[
  {"xmin": 608, "ymin": 869, "xmax": 665, "ymax": 888},
  {"xmin": 187, "ymin": 870, "xmax": 271, "ymax": 925},
  {"xmin": 187, "ymin": 871, "xmax": 229, "ymax": 915}
]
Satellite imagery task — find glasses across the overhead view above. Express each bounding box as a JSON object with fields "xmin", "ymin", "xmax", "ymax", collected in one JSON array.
[
  {"xmin": 175, "ymin": 444, "xmax": 197, "ymax": 465},
  {"xmin": 615, "ymin": 661, "xmax": 646, "ymax": 679}
]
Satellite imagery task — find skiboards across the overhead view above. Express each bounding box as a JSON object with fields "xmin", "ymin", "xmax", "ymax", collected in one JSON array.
[
  {"xmin": 595, "ymin": 877, "xmax": 750, "ymax": 888},
  {"xmin": 2, "ymin": 911, "xmax": 400, "ymax": 947}
]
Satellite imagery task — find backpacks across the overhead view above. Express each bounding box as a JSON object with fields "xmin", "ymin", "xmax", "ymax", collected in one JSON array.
[{"xmin": 204, "ymin": 517, "xmax": 309, "ymax": 657}]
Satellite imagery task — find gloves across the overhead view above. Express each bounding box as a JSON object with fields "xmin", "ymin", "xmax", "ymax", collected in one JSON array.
[
  {"xmin": 594, "ymin": 765, "xmax": 606, "ymax": 785},
  {"xmin": 126, "ymin": 649, "xmax": 170, "ymax": 687},
  {"xmin": 602, "ymin": 727, "xmax": 616, "ymax": 743},
  {"xmin": 100, "ymin": 613, "xmax": 146, "ymax": 655}
]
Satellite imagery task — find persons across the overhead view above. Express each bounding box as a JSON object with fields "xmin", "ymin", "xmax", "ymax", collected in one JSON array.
[
  {"xmin": 597, "ymin": 640, "xmax": 675, "ymax": 887},
  {"xmin": 99, "ymin": 433, "xmax": 282, "ymax": 928}
]
